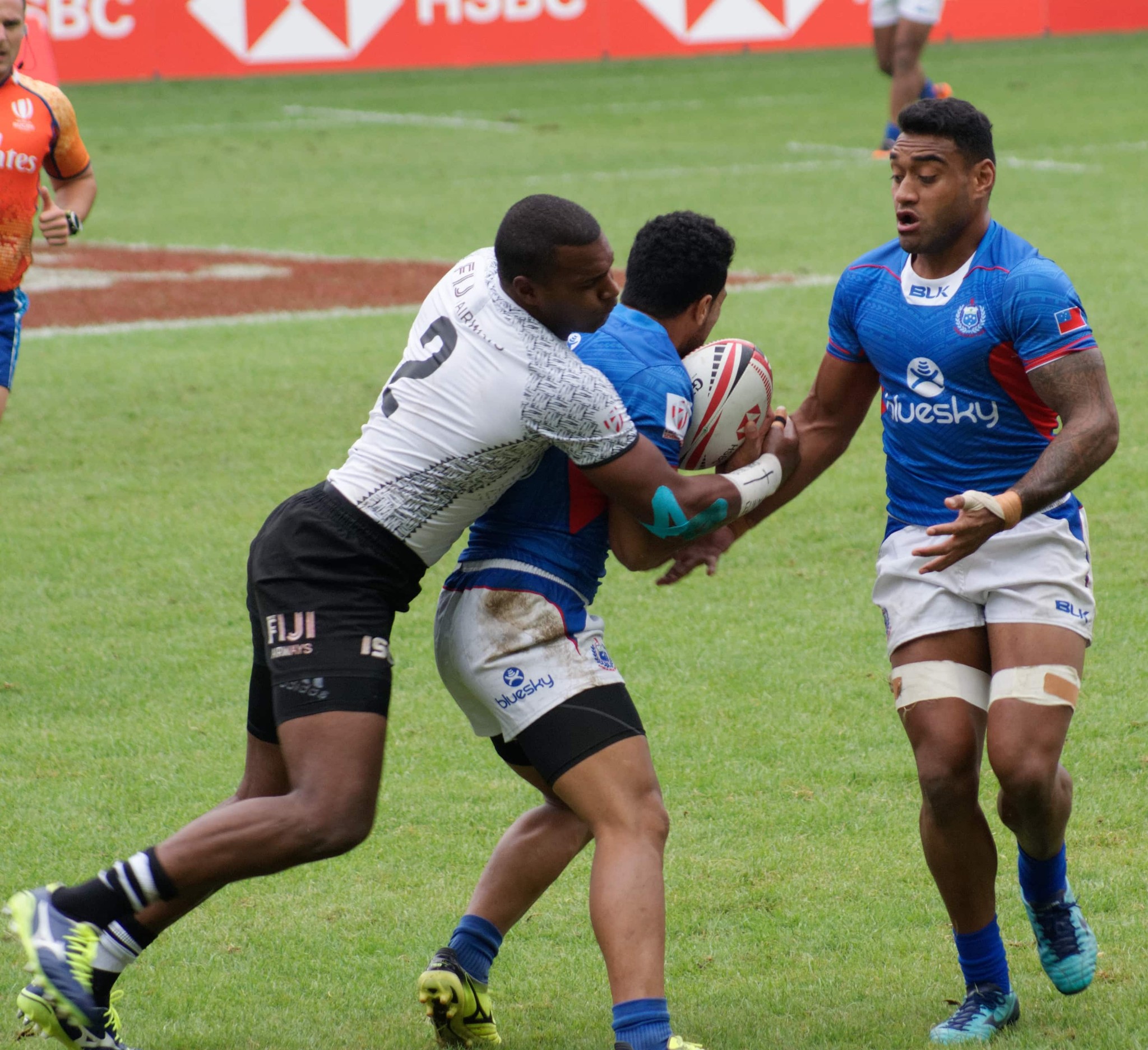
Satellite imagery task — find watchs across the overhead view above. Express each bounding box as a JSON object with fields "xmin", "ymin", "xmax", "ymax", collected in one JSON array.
[{"xmin": 65, "ymin": 212, "xmax": 79, "ymax": 235}]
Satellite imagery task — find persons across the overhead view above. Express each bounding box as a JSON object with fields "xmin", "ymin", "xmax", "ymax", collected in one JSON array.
[
  {"xmin": 420, "ymin": 210, "xmax": 776, "ymax": 1050},
  {"xmin": 0, "ymin": 194, "xmax": 801, "ymax": 1050},
  {"xmin": 870, "ymin": 0, "xmax": 953, "ymax": 160},
  {"xmin": 658, "ymin": 100, "xmax": 1120, "ymax": 1042},
  {"xmin": 0, "ymin": 0, "xmax": 98, "ymax": 417}
]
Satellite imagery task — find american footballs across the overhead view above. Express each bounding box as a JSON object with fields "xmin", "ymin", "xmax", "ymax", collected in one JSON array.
[{"xmin": 674, "ymin": 338, "xmax": 774, "ymax": 471}]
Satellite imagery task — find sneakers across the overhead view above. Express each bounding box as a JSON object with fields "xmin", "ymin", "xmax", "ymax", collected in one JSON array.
[
  {"xmin": 17, "ymin": 974, "xmax": 129, "ymax": 1050},
  {"xmin": 8, "ymin": 881, "xmax": 107, "ymax": 1042},
  {"xmin": 417, "ymin": 947, "xmax": 502, "ymax": 1047},
  {"xmin": 1021, "ymin": 875, "xmax": 1096, "ymax": 994},
  {"xmin": 921, "ymin": 78, "xmax": 952, "ymax": 99},
  {"xmin": 872, "ymin": 121, "xmax": 901, "ymax": 158},
  {"xmin": 930, "ymin": 983, "xmax": 1020, "ymax": 1043}
]
